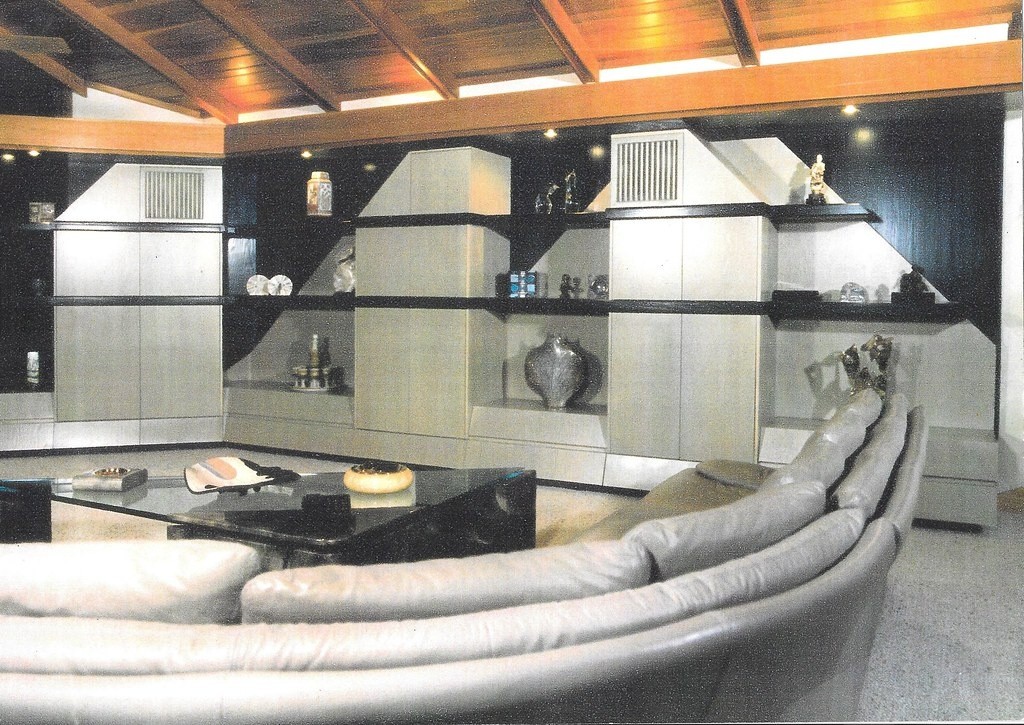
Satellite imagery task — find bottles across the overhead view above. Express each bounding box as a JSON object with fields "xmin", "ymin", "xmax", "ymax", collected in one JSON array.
[
  {"xmin": 307, "ymin": 334, "xmax": 324, "ymax": 387},
  {"xmin": 306, "ymin": 171, "xmax": 332, "ymax": 216},
  {"xmin": 26, "ymin": 352, "xmax": 39, "ymax": 390},
  {"xmin": 524, "ymin": 320, "xmax": 583, "ymax": 410}
]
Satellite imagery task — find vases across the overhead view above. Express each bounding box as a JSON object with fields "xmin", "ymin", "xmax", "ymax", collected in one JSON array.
[{"xmin": 524, "ymin": 332, "xmax": 590, "ymax": 409}]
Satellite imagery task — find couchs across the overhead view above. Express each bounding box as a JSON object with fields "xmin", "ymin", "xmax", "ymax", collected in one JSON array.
[{"xmin": 0, "ymin": 388, "xmax": 929, "ymax": 725}]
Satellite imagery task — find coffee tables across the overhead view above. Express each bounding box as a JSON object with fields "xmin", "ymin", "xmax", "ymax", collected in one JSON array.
[{"xmin": 37, "ymin": 466, "xmax": 538, "ymax": 572}]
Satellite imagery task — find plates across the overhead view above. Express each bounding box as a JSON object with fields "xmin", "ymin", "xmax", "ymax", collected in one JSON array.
[{"xmin": 293, "ymin": 388, "xmax": 331, "ymax": 395}]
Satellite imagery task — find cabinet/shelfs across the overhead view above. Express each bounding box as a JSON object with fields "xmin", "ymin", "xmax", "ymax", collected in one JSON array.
[{"xmin": 0, "ymin": 116, "xmax": 1000, "ymax": 532}]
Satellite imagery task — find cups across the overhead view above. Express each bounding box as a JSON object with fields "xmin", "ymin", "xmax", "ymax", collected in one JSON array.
[
  {"xmin": 291, "ymin": 366, "xmax": 330, "ymax": 389},
  {"xmin": 29, "ymin": 202, "xmax": 55, "ymax": 224}
]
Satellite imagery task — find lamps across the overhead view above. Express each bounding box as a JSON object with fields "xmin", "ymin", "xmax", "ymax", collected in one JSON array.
[{"xmin": 0, "ymin": 18, "xmax": 93, "ymax": 55}]
